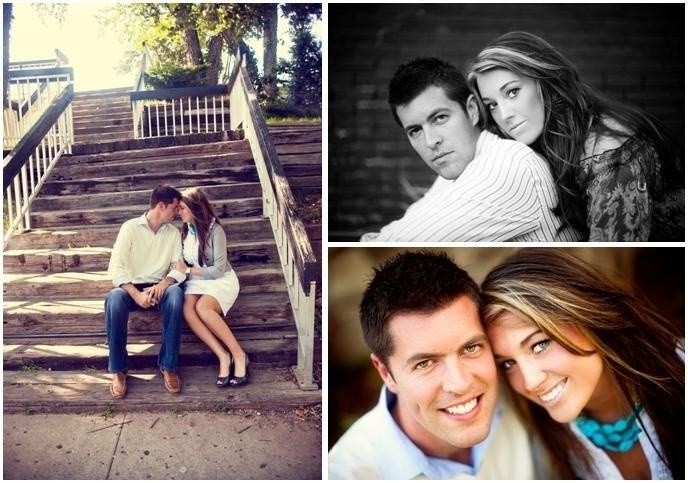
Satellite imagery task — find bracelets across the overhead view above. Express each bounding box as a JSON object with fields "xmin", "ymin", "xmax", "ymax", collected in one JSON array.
[{"xmin": 185, "ymin": 266, "xmax": 192, "ymax": 274}]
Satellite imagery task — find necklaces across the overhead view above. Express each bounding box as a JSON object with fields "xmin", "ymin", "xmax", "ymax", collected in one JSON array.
[{"xmin": 572, "ymin": 406, "xmax": 645, "ymax": 451}]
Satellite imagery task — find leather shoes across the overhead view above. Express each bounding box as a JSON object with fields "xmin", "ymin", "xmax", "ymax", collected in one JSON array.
[
  {"xmin": 110, "ymin": 372, "xmax": 128, "ymax": 399},
  {"xmin": 159, "ymin": 367, "xmax": 183, "ymax": 395}
]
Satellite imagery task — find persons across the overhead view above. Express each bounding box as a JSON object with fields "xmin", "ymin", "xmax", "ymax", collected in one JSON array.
[
  {"xmin": 474, "ymin": 246, "xmax": 685, "ymax": 480},
  {"xmin": 103, "ymin": 184, "xmax": 186, "ymax": 398},
  {"xmin": 463, "ymin": 30, "xmax": 683, "ymax": 241},
  {"xmin": 353, "ymin": 55, "xmax": 588, "ymax": 242},
  {"xmin": 323, "ymin": 249, "xmax": 570, "ymax": 480},
  {"xmin": 175, "ymin": 189, "xmax": 253, "ymax": 388}
]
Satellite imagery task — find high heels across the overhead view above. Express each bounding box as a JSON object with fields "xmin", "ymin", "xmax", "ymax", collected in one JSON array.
[{"xmin": 215, "ymin": 353, "xmax": 251, "ymax": 388}]
